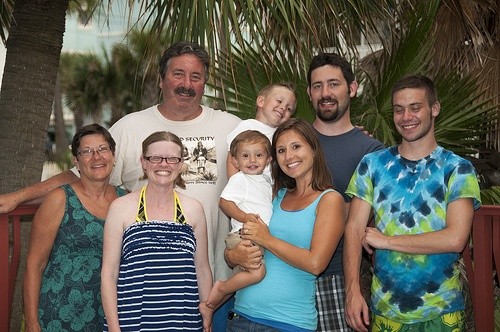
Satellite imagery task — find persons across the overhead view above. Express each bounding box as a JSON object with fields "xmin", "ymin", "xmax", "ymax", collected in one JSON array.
[
  {"xmin": 226, "ymin": 82, "xmax": 297, "ymax": 178},
  {"xmin": 1, "ymin": 40, "xmax": 372, "ymax": 332},
  {"xmin": 101, "ymin": 131, "xmax": 214, "ymax": 332},
  {"xmin": 22, "ymin": 124, "xmax": 131, "ymax": 331},
  {"xmin": 226, "ymin": 117, "xmax": 348, "ymax": 332},
  {"xmin": 342, "ymin": 74, "xmax": 482, "ymax": 332},
  {"xmin": 198, "ymin": 131, "xmax": 273, "ymax": 332},
  {"xmin": 300, "ymin": 53, "xmax": 387, "ymax": 331}
]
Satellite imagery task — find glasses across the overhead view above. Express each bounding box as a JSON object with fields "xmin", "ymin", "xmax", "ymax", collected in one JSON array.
[
  {"xmin": 77, "ymin": 144, "xmax": 113, "ymax": 159},
  {"xmin": 146, "ymin": 156, "xmax": 184, "ymax": 164}
]
